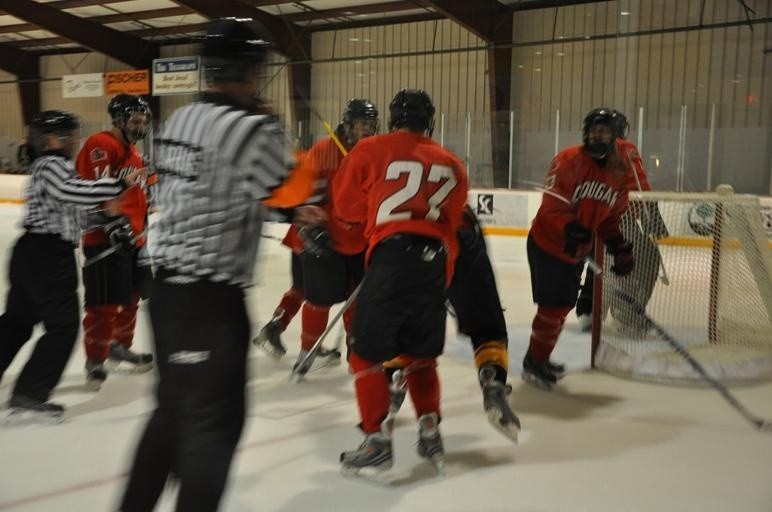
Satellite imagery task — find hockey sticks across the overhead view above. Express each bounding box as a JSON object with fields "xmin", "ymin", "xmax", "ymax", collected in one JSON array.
[
  {"xmin": 584, "ymin": 254, "xmax": 772, "ymax": 434},
  {"xmin": 625, "ymin": 151, "xmax": 670, "ymax": 287},
  {"xmin": 285, "ymin": 276, "xmax": 366, "ymax": 383}
]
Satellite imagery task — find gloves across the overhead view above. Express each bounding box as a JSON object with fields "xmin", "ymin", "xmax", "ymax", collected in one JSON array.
[
  {"xmin": 562, "ymin": 222, "xmax": 592, "ymax": 257},
  {"xmin": 102, "ymin": 213, "xmax": 136, "ymax": 250},
  {"xmin": 605, "ymin": 238, "xmax": 635, "ymax": 278}
]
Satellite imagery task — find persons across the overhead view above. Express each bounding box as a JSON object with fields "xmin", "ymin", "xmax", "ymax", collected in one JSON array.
[
  {"xmin": 329, "ymin": 91, "xmax": 468, "ymax": 468},
  {"xmin": 251, "ymin": 100, "xmax": 377, "ymax": 357},
  {"xmin": 521, "ymin": 108, "xmax": 635, "ymax": 385},
  {"xmin": 77, "ymin": 93, "xmax": 156, "ymax": 375},
  {"xmin": 577, "ymin": 110, "xmax": 669, "ymax": 325},
  {"xmin": 0, "ymin": 111, "xmax": 148, "ymax": 411},
  {"xmin": 118, "ymin": 21, "xmax": 328, "ymax": 511},
  {"xmin": 383, "ymin": 205, "xmax": 526, "ymax": 429}
]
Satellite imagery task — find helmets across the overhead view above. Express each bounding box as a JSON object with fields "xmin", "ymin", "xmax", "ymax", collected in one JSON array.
[
  {"xmin": 200, "ymin": 15, "xmax": 273, "ymax": 84},
  {"xmin": 618, "ymin": 113, "xmax": 629, "ymax": 139},
  {"xmin": 107, "ymin": 92, "xmax": 154, "ymax": 146},
  {"xmin": 338, "ymin": 97, "xmax": 379, "ymax": 143},
  {"xmin": 387, "ymin": 87, "xmax": 437, "ymax": 136},
  {"xmin": 27, "ymin": 109, "xmax": 79, "ymax": 158},
  {"xmin": 581, "ymin": 108, "xmax": 618, "ymax": 160}
]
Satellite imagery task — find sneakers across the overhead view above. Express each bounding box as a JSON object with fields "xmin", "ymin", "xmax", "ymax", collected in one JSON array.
[
  {"xmin": 549, "ymin": 363, "xmax": 565, "ymax": 374},
  {"xmin": 415, "ymin": 412, "xmax": 443, "ymax": 459},
  {"xmin": 479, "ymin": 366, "xmax": 520, "ymax": 427},
  {"xmin": 291, "ymin": 348, "xmax": 340, "ymax": 373},
  {"xmin": 110, "ymin": 342, "xmax": 153, "ymax": 364},
  {"xmin": 523, "ymin": 349, "xmax": 557, "ymax": 385},
  {"xmin": 86, "ymin": 358, "xmax": 108, "ymax": 380},
  {"xmin": 10, "ymin": 394, "xmax": 64, "ymax": 411},
  {"xmin": 260, "ymin": 319, "xmax": 286, "ymax": 352},
  {"xmin": 340, "ymin": 432, "xmax": 393, "ymax": 467}
]
